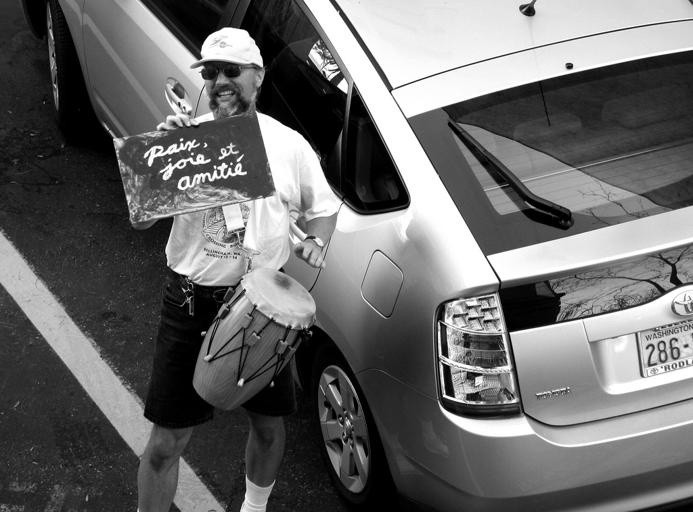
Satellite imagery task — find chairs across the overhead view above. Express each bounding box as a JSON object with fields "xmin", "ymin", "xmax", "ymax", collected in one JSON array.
[{"xmin": 513, "ymin": 83, "xmax": 693, "ymax": 165}]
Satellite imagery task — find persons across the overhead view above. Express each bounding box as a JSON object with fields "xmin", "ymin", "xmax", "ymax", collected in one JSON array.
[{"xmin": 127, "ymin": 24, "xmax": 344, "ymax": 512}]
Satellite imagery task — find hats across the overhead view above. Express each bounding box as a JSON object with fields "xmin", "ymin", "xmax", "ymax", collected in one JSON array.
[{"xmin": 189, "ymin": 26, "xmax": 263, "ymax": 70}]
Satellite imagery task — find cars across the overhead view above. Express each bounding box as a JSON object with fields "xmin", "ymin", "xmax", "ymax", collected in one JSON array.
[{"xmin": 19, "ymin": 0, "xmax": 693, "ymax": 512}]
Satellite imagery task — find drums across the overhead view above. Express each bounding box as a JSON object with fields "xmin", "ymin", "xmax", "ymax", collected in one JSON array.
[{"xmin": 192, "ymin": 268, "xmax": 316, "ymax": 410}]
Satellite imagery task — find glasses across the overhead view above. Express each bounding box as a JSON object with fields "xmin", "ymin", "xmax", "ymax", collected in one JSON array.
[{"xmin": 199, "ymin": 67, "xmax": 260, "ymax": 80}]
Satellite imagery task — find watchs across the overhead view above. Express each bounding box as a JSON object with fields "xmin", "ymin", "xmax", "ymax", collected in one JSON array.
[{"xmin": 301, "ymin": 234, "xmax": 325, "ymax": 253}]
